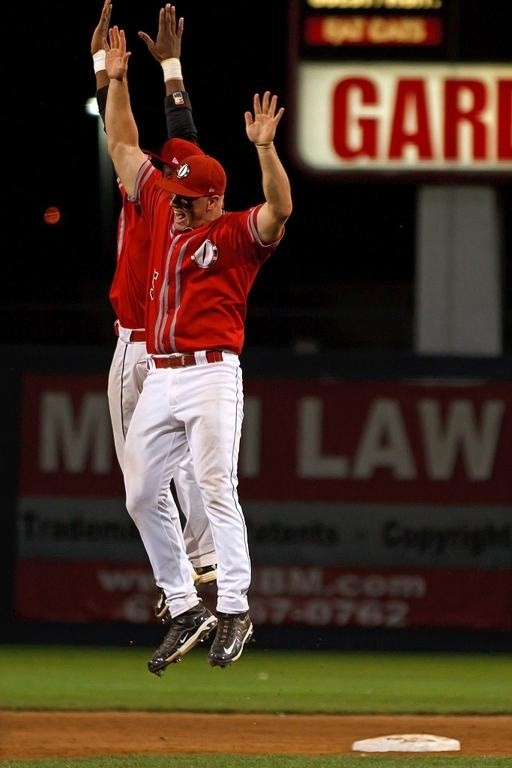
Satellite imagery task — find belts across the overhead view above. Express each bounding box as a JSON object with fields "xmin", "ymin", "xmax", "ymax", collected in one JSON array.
[
  {"xmin": 114, "ymin": 321, "xmax": 146, "ymax": 342},
  {"xmin": 147, "ymin": 350, "xmax": 222, "ymax": 369}
]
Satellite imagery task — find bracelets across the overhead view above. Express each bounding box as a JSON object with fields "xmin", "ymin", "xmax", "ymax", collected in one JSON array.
[
  {"xmin": 92, "ymin": 49, "xmax": 107, "ymax": 73},
  {"xmin": 160, "ymin": 57, "xmax": 185, "ymax": 82}
]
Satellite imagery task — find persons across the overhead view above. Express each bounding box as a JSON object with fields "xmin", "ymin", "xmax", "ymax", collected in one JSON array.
[
  {"xmin": 90, "ymin": 0, "xmax": 218, "ymax": 624},
  {"xmin": 101, "ymin": 24, "xmax": 295, "ymax": 676}
]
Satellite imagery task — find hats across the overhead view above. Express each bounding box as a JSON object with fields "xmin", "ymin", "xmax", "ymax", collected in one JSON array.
[
  {"xmin": 154, "ymin": 156, "xmax": 227, "ymax": 199},
  {"xmin": 141, "ymin": 138, "xmax": 205, "ymax": 170}
]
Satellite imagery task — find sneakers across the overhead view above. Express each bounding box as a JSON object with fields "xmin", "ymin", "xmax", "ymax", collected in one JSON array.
[
  {"xmin": 194, "ymin": 564, "xmax": 217, "ymax": 583},
  {"xmin": 148, "ymin": 602, "xmax": 218, "ymax": 677},
  {"xmin": 208, "ymin": 611, "xmax": 254, "ymax": 668},
  {"xmin": 154, "ymin": 568, "xmax": 201, "ymax": 623}
]
